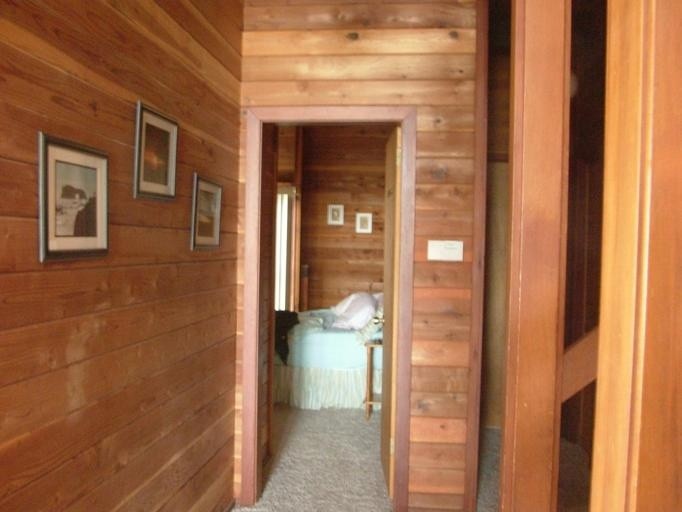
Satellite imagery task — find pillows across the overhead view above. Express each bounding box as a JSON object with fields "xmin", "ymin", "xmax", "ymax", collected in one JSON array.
[{"xmin": 331, "ymin": 288, "xmax": 389, "ymax": 333}]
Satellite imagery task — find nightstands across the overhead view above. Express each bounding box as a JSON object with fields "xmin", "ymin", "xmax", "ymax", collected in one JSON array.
[{"xmin": 360, "ymin": 340, "xmax": 381, "ymax": 421}]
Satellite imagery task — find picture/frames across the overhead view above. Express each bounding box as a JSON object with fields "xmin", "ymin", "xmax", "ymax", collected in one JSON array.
[
  {"xmin": 328, "ymin": 204, "xmax": 345, "ymax": 227},
  {"xmin": 132, "ymin": 98, "xmax": 180, "ymax": 204},
  {"xmin": 188, "ymin": 168, "xmax": 224, "ymax": 253},
  {"xmin": 355, "ymin": 211, "xmax": 373, "ymax": 234},
  {"xmin": 36, "ymin": 128, "xmax": 111, "ymax": 265}
]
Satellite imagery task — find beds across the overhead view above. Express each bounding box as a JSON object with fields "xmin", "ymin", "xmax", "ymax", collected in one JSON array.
[{"xmin": 269, "ymin": 300, "xmax": 399, "ymax": 416}]
